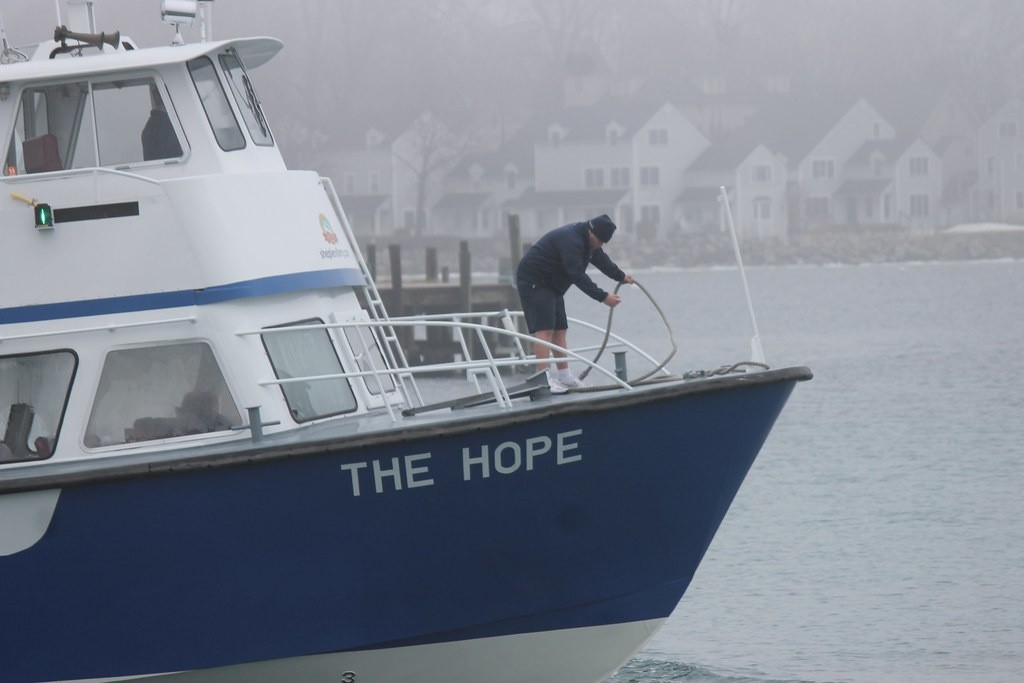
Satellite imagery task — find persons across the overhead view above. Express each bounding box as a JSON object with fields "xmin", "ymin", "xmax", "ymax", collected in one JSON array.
[{"xmin": 516, "ymin": 215, "xmax": 634, "ymax": 394}]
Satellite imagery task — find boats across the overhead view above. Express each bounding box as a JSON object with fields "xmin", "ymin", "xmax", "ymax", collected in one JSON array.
[{"xmin": 0, "ymin": 1, "xmax": 812, "ymax": 682}]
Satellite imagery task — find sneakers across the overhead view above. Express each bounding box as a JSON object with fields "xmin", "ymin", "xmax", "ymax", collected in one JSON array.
[{"xmin": 549, "ymin": 377, "xmax": 584, "ymax": 393}]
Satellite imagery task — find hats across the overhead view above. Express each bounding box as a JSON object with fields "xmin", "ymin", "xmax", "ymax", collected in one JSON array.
[{"xmin": 590, "ymin": 214, "xmax": 616, "ymax": 243}]
